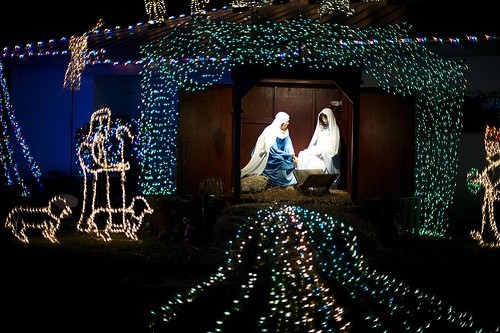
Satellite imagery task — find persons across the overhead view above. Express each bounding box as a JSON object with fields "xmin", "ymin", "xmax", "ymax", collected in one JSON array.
[
  {"xmin": 298, "ymin": 107, "xmax": 341, "ymax": 190},
  {"xmin": 240, "ymin": 111, "xmax": 298, "ymax": 187}
]
